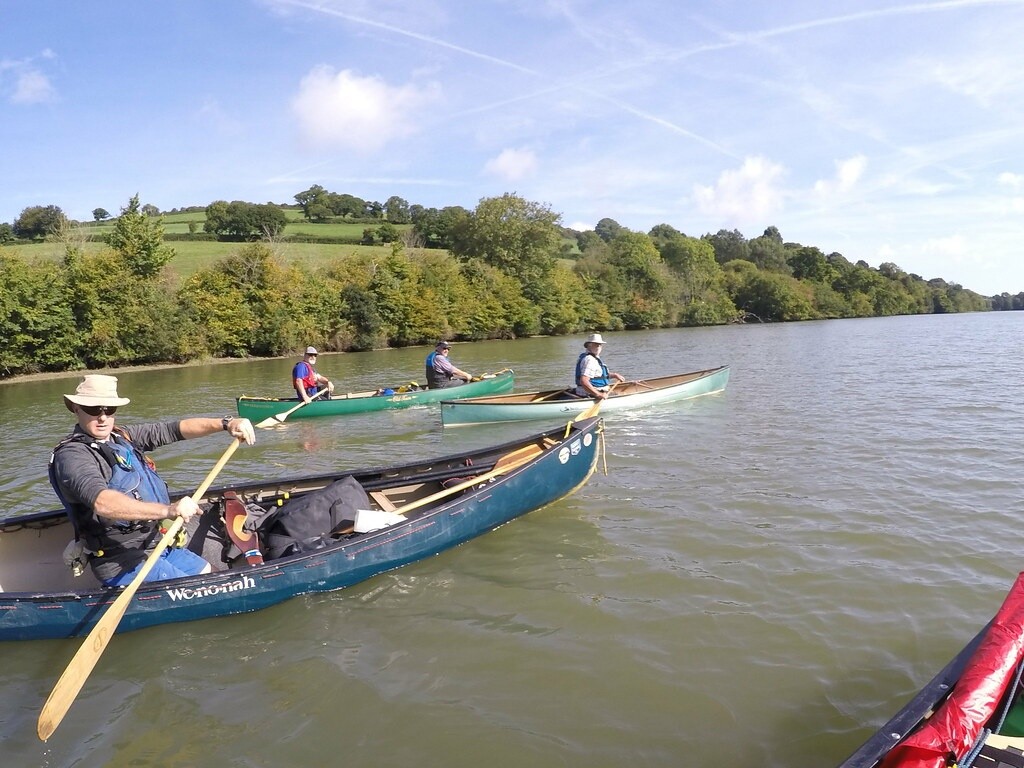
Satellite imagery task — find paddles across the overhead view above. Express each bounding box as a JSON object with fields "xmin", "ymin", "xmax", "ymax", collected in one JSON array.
[
  {"xmin": 573, "ymin": 379, "xmax": 622, "ymax": 422},
  {"xmin": 254, "ymin": 387, "xmax": 330, "ymax": 430},
  {"xmin": 339, "ymin": 443, "xmax": 543, "ymax": 536},
  {"xmin": 36, "ymin": 436, "xmax": 245, "ymax": 743}
]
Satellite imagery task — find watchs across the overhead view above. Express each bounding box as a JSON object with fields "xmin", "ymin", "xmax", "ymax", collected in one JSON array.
[{"xmin": 222, "ymin": 415, "xmax": 233, "ymax": 430}]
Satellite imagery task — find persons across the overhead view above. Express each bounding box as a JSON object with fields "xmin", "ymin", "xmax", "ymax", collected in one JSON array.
[
  {"xmin": 575, "ymin": 334, "xmax": 626, "ymax": 400},
  {"xmin": 293, "ymin": 346, "xmax": 334, "ymax": 405},
  {"xmin": 426, "ymin": 342, "xmax": 473, "ymax": 389},
  {"xmin": 48, "ymin": 374, "xmax": 256, "ymax": 586}
]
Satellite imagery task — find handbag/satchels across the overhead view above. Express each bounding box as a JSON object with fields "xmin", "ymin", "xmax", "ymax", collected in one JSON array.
[{"xmin": 258, "ymin": 473, "xmax": 373, "ymax": 560}]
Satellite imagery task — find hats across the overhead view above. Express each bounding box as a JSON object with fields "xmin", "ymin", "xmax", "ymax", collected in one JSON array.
[
  {"xmin": 305, "ymin": 346, "xmax": 319, "ymax": 355},
  {"xmin": 63, "ymin": 375, "xmax": 131, "ymax": 414},
  {"xmin": 583, "ymin": 334, "xmax": 607, "ymax": 348},
  {"xmin": 436, "ymin": 341, "xmax": 452, "ymax": 349}
]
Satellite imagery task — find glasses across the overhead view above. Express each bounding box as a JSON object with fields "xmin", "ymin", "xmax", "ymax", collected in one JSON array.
[{"xmin": 79, "ymin": 404, "xmax": 117, "ymax": 415}]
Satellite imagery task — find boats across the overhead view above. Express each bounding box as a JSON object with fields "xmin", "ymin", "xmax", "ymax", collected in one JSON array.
[
  {"xmin": 439, "ymin": 363, "xmax": 734, "ymax": 425},
  {"xmin": 237, "ymin": 368, "xmax": 516, "ymax": 424},
  {"xmin": 0, "ymin": 419, "xmax": 608, "ymax": 643}
]
